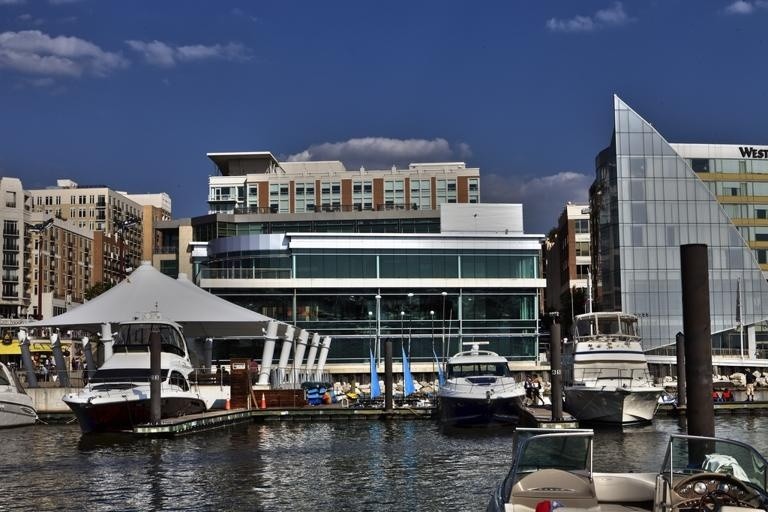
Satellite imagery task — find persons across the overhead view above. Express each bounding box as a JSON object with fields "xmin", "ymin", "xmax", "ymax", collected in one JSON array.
[
  {"xmin": 744, "ymin": 368, "xmax": 757, "ymax": 402},
  {"xmin": 712, "ymin": 387, "xmax": 721, "ymax": 402},
  {"xmin": 722, "ymin": 387, "xmax": 734, "ymax": 402},
  {"xmin": 531, "ymin": 374, "xmax": 544, "ymax": 407},
  {"xmin": 523, "ymin": 375, "xmax": 533, "ymax": 405}
]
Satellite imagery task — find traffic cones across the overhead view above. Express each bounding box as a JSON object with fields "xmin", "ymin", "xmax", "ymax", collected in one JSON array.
[
  {"xmin": 259, "ymin": 393, "xmax": 267, "ymax": 410},
  {"xmin": 224, "ymin": 393, "xmax": 230, "ymax": 410}
]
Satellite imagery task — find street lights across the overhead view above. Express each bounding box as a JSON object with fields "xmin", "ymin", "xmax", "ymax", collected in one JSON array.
[
  {"xmin": 23, "ymin": 218, "xmax": 55, "ymax": 336},
  {"xmin": 111, "ymin": 216, "xmax": 142, "ymax": 282}
]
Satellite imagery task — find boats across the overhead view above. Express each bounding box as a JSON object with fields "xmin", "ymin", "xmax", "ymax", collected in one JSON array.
[
  {"xmin": 0, "ymin": 360, "xmax": 38, "ymax": 429},
  {"xmin": 561, "ymin": 272, "xmax": 665, "ymax": 427},
  {"xmin": 312, "ymin": 295, "xmax": 530, "ymax": 360},
  {"xmin": 712, "ymin": 376, "xmax": 735, "ymax": 404},
  {"xmin": 60, "ymin": 295, "xmax": 235, "ymax": 434},
  {"xmin": 434, "ymin": 288, "xmax": 527, "ymax": 429},
  {"xmin": 656, "ymin": 380, "xmax": 686, "ymax": 406},
  {"xmin": 485, "ymin": 427, "xmax": 766, "ymax": 512}
]
